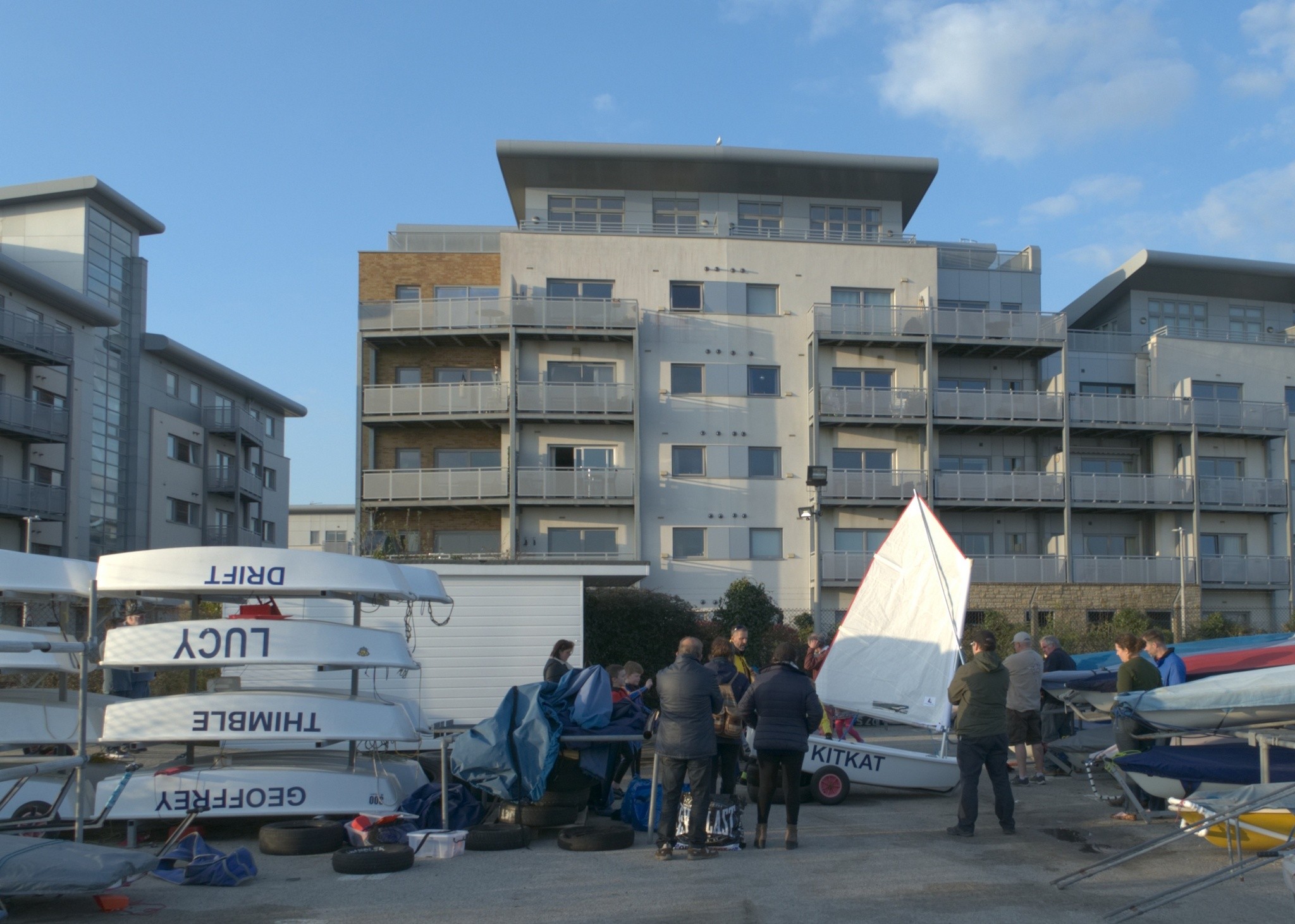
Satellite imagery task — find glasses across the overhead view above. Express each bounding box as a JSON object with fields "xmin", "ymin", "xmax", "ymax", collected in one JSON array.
[
  {"xmin": 731, "ymin": 625, "xmax": 745, "ymax": 635},
  {"xmin": 1041, "ymin": 643, "xmax": 1051, "ymax": 650}
]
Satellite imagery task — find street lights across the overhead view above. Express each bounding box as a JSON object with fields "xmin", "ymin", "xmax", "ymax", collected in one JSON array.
[
  {"xmin": 21, "ymin": 516, "xmax": 41, "ymax": 628},
  {"xmin": 1170, "ymin": 527, "xmax": 1188, "ymax": 641}
]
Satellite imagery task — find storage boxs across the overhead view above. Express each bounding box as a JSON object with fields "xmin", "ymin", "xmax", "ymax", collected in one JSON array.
[
  {"xmin": 407, "ymin": 829, "xmax": 469, "ymax": 860},
  {"xmin": 169, "ymin": 824, "xmax": 205, "ymax": 842},
  {"xmin": 343, "ymin": 817, "xmax": 416, "ymax": 847}
]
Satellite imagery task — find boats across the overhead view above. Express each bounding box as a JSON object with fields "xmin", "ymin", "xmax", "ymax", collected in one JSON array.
[
  {"xmin": 1, "ymin": 545, "xmax": 455, "ymax": 846},
  {"xmin": 1035, "ymin": 628, "xmax": 1295, "ymax": 789}
]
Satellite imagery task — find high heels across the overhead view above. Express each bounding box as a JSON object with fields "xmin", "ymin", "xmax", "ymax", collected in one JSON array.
[
  {"xmin": 786, "ymin": 824, "xmax": 798, "ymax": 849},
  {"xmin": 754, "ymin": 823, "xmax": 767, "ymax": 849}
]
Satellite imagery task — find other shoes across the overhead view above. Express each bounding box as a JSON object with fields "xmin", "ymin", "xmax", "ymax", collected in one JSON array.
[
  {"xmin": 1007, "ymin": 765, "xmax": 1015, "ymax": 773},
  {"xmin": 1109, "ymin": 796, "xmax": 1130, "ymax": 807},
  {"xmin": 1111, "ymin": 813, "xmax": 1136, "ymax": 820},
  {"xmin": 826, "ymin": 732, "xmax": 833, "ymax": 740}
]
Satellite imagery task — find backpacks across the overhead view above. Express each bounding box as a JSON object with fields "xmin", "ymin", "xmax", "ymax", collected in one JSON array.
[{"xmin": 712, "ymin": 670, "xmax": 743, "ymax": 737}]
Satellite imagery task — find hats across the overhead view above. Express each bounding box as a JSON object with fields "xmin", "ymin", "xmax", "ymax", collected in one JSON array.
[
  {"xmin": 970, "ymin": 630, "xmax": 995, "ymax": 645},
  {"xmin": 1010, "ymin": 631, "xmax": 1032, "ymax": 644}
]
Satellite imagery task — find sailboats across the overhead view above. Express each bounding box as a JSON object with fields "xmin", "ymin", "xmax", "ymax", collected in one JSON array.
[{"xmin": 744, "ymin": 490, "xmax": 973, "ymax": 806}]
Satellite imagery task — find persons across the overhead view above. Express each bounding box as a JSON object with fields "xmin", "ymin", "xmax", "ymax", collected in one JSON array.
[
  {"xmin": 543, "ymin": 640, "xmax": 575, "ymax": 683},
  {"xmin": 1141, "ymin": 627, "xmax": 1185, "ymax": 819},
  {"xmin": 1110, "ymin": 633, "xmax": 1163, "ymax": 820},
  {"xmin": 739, "ymin": 640, "xmax": 823, "ymax": 849},
  {"xmin": 621, "ymin": 662, "xmax": 659, "ymax": 780},
  {"xmin": 728, "ymin": 624, "xmax": 756, "ymax": 704},
  {"xmin": 1003, "ymin": 631, "xmax": 1046, "ymax": 787},
  {"xmin": 833, "ymin": 707, "xmax": 865, "ymax": 743},
  {"xmin": 805, "ymin": 633, "xmax": 833, "ymax": 736},
  {"xmin": 607, "ymin": 665, "xmax": 635, "ymax": 800},
  {"xmin": 704, "ymin": 637, "xmax": 751, "ymax": 794},
  {"xmin": 1039, "ymin": 635, "xmax": 1077, "ymax": 775},
  {"xmin": 947, "ymin": 631, "xmax": 1017, "ymax": 838},
  {"xmin": 657, "ymin": 637, "xmax": 725, "ymax": 860}
]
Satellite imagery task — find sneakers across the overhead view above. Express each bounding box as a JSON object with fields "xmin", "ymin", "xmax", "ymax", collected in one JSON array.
[
  {"xmin": 1002, "ymin": 826, "xmax": 1016, "ymax": 835},
  {"xmin": 613, "ymin": 788, "xmax": 626, "ymax": 797},
  {"xmin": 688, "ymin": 845, "xmax": 718, "ymax": 858},
  {"xmin": 1029, "ymin": 773, "xmax": 1046, "ymax": 784},
  {"xmin": 612, "ymin": 794, "xmax": 622, "ymax": 800},
  {"xmin": 946, "ymin": 824, "xmax": 974, "ymax": 837},
  {"xmin": 655, "ymin": 848, "xmax": 673, "ymax": 860},
  {"xmin": 1009, "ymin": 775, "xmax": 1030, "ymax": 786}
]
